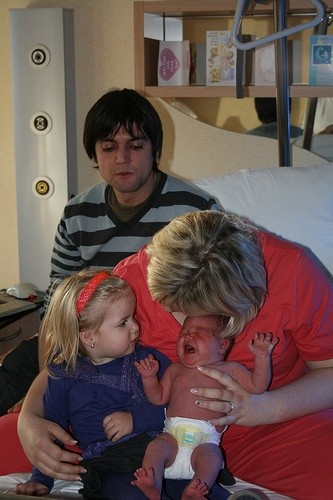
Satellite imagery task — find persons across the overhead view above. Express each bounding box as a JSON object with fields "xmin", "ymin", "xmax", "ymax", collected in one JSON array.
[
  {"xmin": 132, "ymin": 314, "xmax": 279, "ymax": 500},
  {"xmin": 14, "ymin": 266, "xmax": 233, "ymax": 500},
  {"xmin": 243, "ymin": 97, "xmax": 305, "ymax": 140},
  {"xmin": 18, "ymin": 210, "xmax": 333, "ymax": 500},
  {"xmin": 0, "ymin": 88, "xmax": 223, "ymax": 416}
]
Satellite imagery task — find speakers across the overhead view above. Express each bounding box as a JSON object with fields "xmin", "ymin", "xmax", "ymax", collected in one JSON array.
[{"xmin": 9, "ymin": 6, "xmax": 76, "ymax": 291}]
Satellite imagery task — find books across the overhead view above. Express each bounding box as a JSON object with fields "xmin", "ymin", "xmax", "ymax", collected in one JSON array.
[{"xmin": 157, "ymin": 30, "xmax": 333, "ymax": 86}]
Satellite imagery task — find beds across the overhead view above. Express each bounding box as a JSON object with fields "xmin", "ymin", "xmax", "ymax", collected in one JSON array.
[{"xmin": 0, "ymin": 162, "xmax": 333, "ymax": 500}]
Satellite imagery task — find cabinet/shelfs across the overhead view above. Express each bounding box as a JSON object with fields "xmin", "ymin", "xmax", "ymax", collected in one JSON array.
[{"xmin": 133, "ymin": 0, "xmax": 333, "ymax": 98}]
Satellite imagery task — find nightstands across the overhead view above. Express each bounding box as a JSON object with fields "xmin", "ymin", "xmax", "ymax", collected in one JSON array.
[{"xmin": 0, "ymin": 289, "xmax": 47, "ymax": 355}]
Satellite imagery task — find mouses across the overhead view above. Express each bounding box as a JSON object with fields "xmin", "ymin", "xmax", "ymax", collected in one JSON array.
[{"xmin": 6, "ymin": 284, "xmax": 38, "ymax": 300}]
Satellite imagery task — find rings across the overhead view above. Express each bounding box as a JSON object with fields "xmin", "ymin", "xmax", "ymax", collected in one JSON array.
[{"xmin": 225, "ymin": 401, "xmax": 233, "ymax": 414}]
